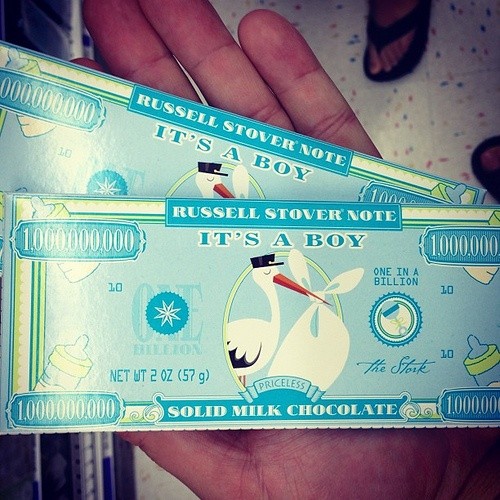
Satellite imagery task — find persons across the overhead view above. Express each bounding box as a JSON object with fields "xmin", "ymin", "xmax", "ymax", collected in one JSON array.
[
  {"xmin": 360, "ymin": 0, "xmax": 432, "ymax": 82},
  {"xmin": 63, "ymin": 0, "xmax": 500, "ymax": 500}
]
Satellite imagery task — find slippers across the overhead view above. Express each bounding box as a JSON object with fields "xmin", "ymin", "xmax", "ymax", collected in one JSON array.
[
  {"xmin": 364, "ymin": 0, "xmax": 432, "ymax": 81},
  {"xmin": 471, "ymin": 134, "xmax": 499, "ymax": 206}
]
